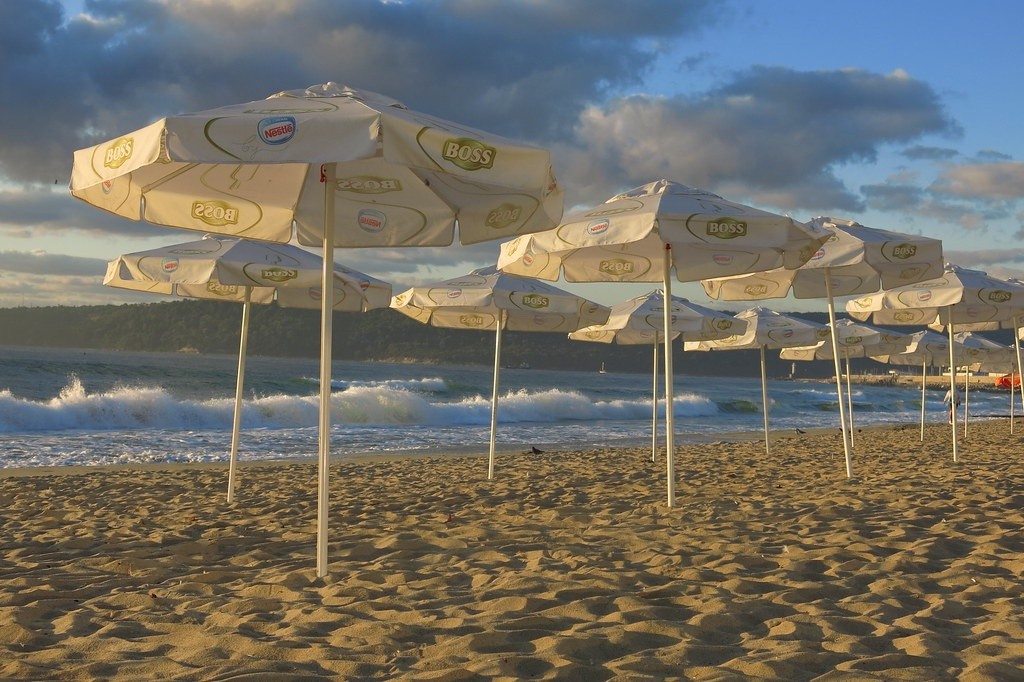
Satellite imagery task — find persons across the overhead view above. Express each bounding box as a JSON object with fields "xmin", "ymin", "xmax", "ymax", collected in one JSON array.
[{"xmin": 944, "ymin": 389, "xmax": 961, "ymax": 425}]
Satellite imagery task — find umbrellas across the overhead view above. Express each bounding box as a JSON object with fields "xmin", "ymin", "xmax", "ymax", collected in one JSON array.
[
  {"xmin": 779, "ymin": 318, "xmax": 913, "ymax": 447},
  {"xmin": 72, "ymin": 83, "xmax": 565, "ymax": 577},
  {"xmin": 846, "ymin": 263, "xmax": 1024, "ymax": 462},
  {"xmin": 568, "ymin": 289, "xmax": 747, "ymax": 462},
  {"xmin": 684, "ymin": 307, "xmax": 831, "ymax": 453},
  {"xmin": 701, "ymin": 217, "xmax": 944, "ymax": 477},
  {"xmin": 869, "ymin": 277, "xmax": 1024, "ymax": 441},
  {"xmin": 496, "ymin": 179, "xmax": 835, "ymax": 507},
  {"xmin": 102, "ymin": 236, "xmax": 392, "ymax": 502},
  {"xmin": 389, "ymin": 264, "xmax": 612, "ymax": 480}
]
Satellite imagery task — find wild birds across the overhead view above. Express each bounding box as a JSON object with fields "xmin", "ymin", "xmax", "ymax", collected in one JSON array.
[
  {"xmin": 793, "ymin": 427, "xmax": 807, "ymax": 438},
  {"xmin": 531, "ymin": 446, "xmax": 546, "ymax": 460}
]
